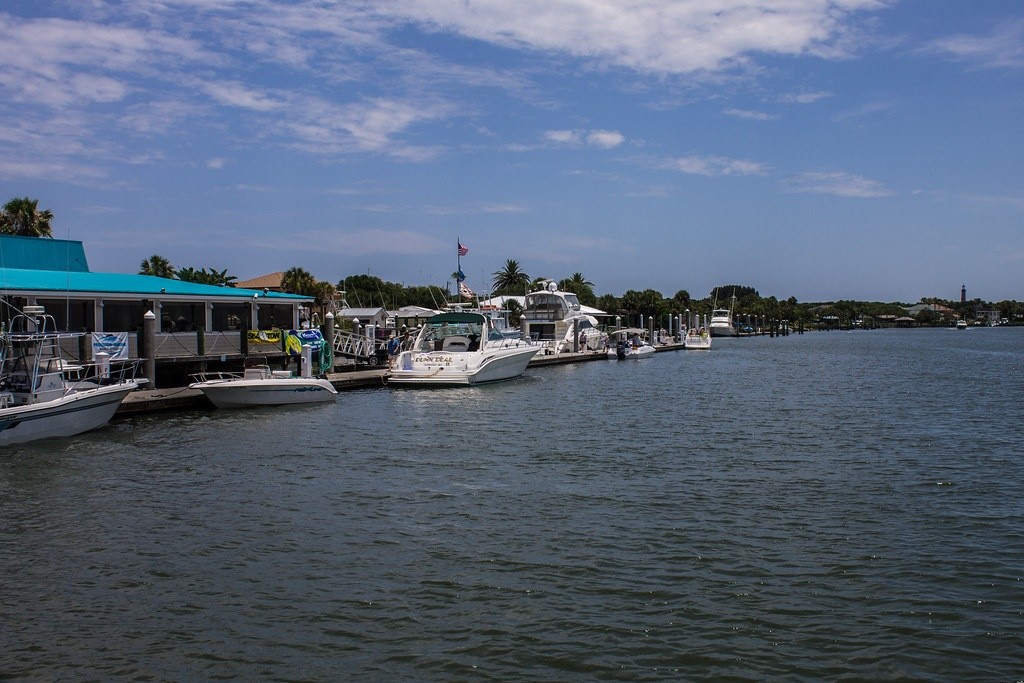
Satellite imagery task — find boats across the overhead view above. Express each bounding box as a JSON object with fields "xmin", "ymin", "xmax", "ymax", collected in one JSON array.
[
  {"xmin": 707, "ymin": 286, "xmax": 736, "ymax": 336},
  {"xmin": 0, "ymin": 299, "xmax": 151, "ymax": 444},
  {"xmin": 956, "ymin": 319, "xmax": 967, "ymax": 329},
  {"xmin": 606, "ymin": 328, "xmax": 656, "ymax": 359},
  {"xmin": 683, "ymin": 327, "xmax": 712, "ymax": 350},
  {"xmin": 384, "ymin": 304, "xmax": 542, "ymax": 386},
  {"xmin": 186, "ymin": 355, "xmax": 338, "ymax": 409}
]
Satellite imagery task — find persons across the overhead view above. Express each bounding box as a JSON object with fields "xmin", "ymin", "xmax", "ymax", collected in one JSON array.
[
  {"xmin": 404, "ymin": 330, "xmax": 414, "ymax": 350},
  {"xmin": 387, "ymin": 334, "xmax": 399, "ymax": 371},
  {"xmin": 580, "ymin": 325, "xmax": 706, "ymax": 353}
]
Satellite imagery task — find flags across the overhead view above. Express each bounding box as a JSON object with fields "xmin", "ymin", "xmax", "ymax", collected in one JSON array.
[
  {"xmin": 458, "ymin": 243, "xmax": 468, "ymax": 256},
  {"xmin": 459, "ymin": 282, "xmax": 475, "ymax": 300},
  {"xmin": 458, "ymin": 269, "xmax": 467, "ymax": 282}
]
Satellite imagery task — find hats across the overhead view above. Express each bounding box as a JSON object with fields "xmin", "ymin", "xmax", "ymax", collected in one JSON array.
[
  {"xmin": 404, "ymin": 330, "xmax": 409, "ymax": 335},
  {"xmin": 389, "ymin": 335, "xmax": 394, "ymax": 339}
]
